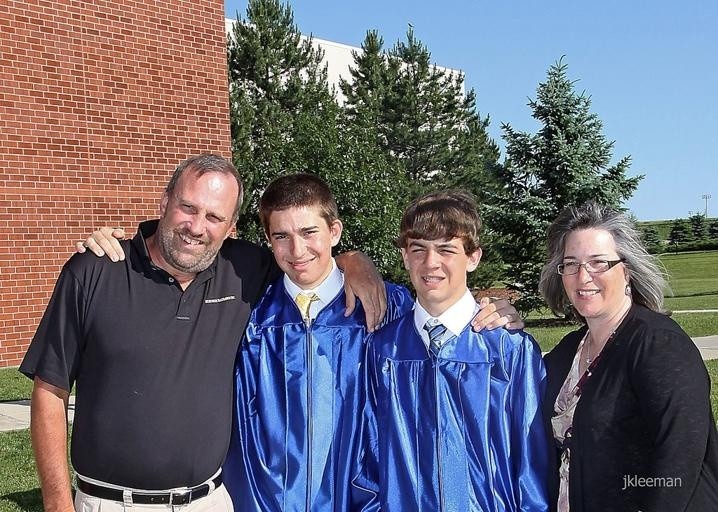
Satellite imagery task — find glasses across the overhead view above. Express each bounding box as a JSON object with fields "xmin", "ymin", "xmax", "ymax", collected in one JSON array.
[{"xmin": 554, "ymin": 258, "xmax": 624, "ymax": 277}]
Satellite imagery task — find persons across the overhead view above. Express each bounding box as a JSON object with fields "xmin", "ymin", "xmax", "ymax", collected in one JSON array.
[
  {"xmin": 76, "ymin": 174, "xmax": 525, "ymax": 511},
  {"xmin": 352, "ymin": 188, "xmax": 554, "ymax": 511},
  {"xmin": 15, "ymin": 152, "xmax": 389, "ymax": 511},
  {"xmin": 536, "ymin": 200, "xmax": 716, "ymax": 512}
]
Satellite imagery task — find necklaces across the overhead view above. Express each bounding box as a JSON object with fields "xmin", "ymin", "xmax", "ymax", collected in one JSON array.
[{"xmin": 584, "ymin": 330, "xmax": 615, "ymax": 376}]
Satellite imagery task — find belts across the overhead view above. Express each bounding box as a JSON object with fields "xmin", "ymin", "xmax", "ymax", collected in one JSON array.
[{"xmin": 78, "ymin": 475, "xmax": 223, "ymax": 506}]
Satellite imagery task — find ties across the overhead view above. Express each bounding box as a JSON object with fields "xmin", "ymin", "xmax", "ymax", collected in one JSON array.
[
  {"xmin": 294, "ymin": 292, "xmax": 316, "ymax": 319},
  {"xmin": 422, "ymin": 323, "xmax": 447, "ymax": 360}
]
